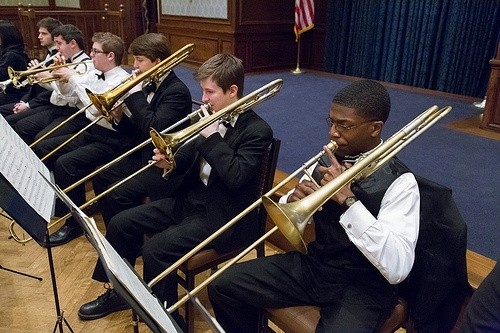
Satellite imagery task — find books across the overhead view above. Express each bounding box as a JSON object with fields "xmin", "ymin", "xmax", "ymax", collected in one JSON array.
[
  {"xmin": 53, "ymin": 184, "xmax": 178, "ymax": 333},
  {"xmin": 0, "ymin": 114, "xmax": 55, "ymax": 223}
]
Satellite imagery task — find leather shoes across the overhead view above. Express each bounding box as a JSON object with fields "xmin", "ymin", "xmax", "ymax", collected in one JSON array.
[
  {"xmin": 43, "ymin": 223, "xmax": 83, "ymax": 246},
  {"xmin": 77, "ymin": 282, "xmax": 131, "ymax": 320}
]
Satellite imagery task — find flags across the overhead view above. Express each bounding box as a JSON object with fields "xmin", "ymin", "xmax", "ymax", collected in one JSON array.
[{"xmin": 294, "ymin": 0, "xmax": 315, "ymax": 43}]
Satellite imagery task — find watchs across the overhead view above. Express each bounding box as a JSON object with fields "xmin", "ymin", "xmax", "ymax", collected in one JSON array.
[{"xmin": 340, "ymin": 196, "xmax": 358, "ymax": 210}]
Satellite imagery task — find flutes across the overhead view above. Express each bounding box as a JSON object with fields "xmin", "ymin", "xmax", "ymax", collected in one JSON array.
[{"xmin": 0, "ymin": 51, "xmax": 62, "ymax": 94}]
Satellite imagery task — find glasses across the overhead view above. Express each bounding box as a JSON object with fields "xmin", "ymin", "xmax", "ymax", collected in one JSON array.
[
  {"xmin": 326, "ymin": 117, "xmax": 376, "ymax": 133},
  {"xmin": 91, "ymin": 48, "xmax": 110, "ymax": 56}
]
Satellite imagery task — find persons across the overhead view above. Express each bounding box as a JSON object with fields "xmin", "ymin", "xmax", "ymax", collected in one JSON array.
[
  {"xmin": 452, "ymin": 258, "xmax": 500, "ymax": 333},
  {"xmin": 0, "ymin": 17, "xmax": 138, "ymax": 163},
  {"xmin": 77, "ymin": 54, "xmax": 274, "ymax": 333},
  {"xmin": 33, "ymin": 33, "xmax": 192, "ymax": 247},
  {"xmin": 206, "ymin": 78, "xmax": 421, "ymax": 333}
]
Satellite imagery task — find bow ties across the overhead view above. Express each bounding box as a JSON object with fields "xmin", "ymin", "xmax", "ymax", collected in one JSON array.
[
  {"xmin": 146, "ymin": 82, "xmax": 157, "ymax": 95},
  {"xmin": 47, "ymin": 49, "xmax": 51, "ymax": 55},
  {"xmin": 222, "ymin": 121, "xmax": 229, "ymax": 129},
  {"xmin": 95, "ymin": 72, "xmax": 105, "ymax": 80},
  {"xmin": 65, "ymin": 50, "xmax": 85, "ymax": 63}
]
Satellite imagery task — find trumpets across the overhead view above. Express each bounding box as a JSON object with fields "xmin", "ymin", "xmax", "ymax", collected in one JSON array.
[
  {"xmin": 25, "ymin": 42, "xmax": 197, "ymax": 162},
  {"xmin": 7, "ymin": 59, "xmax": 95, "ymax": 87},
  {"xmin": 147, "ymin": 104, "xmax": 453, "ymax": 313},
  {"xmin": 7, "ymin": 77, "xmax": 285, "ymax": 246}
]
